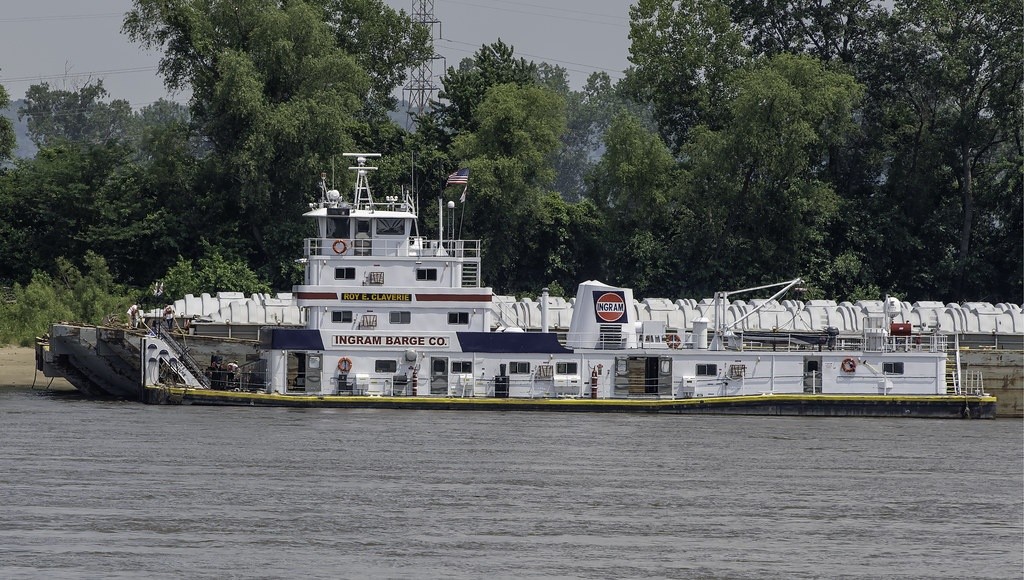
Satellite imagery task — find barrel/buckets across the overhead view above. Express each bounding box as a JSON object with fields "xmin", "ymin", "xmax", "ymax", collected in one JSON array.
[{"xmin": 189, "ymin": 328, "xmax": 195, "ymax": 335}]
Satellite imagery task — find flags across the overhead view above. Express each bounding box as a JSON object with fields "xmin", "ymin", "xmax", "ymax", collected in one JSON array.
[{"xmin": 446, "ymin": 167, "xmax": 469, "ymax": 184}]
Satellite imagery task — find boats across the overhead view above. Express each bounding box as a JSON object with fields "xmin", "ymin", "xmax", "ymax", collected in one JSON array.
[{"xmin": 35, "ymin": 151, "xmax": 998, "ymax": 419}]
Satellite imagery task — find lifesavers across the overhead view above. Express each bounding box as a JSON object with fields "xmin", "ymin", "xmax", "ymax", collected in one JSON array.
[
  {"xmin": 332, "ymin": 240, "xmax": 346, "ymax": 253},
  {"xmin": 338, "ymin": 358, "xmax": 352, "ymax": 371},
  {"xmin": 842, "ymin": 359, "xmax": 856, "ymax": 372},
  {"xmin": 666, "ymin": 335, "xmax": 681, "ymax": 348}
]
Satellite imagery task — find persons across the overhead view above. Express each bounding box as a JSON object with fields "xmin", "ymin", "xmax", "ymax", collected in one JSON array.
[
  {"xmin": 127, "ymin": 302, "xmax": 142, "ymax": 330},
  {"xmin": 163, "ymin": 299, "xmax": 177, "ymax": 329},
  {"xmin": 211, "ymin": 355, "xmax": 241, "ymax": 384}
]
